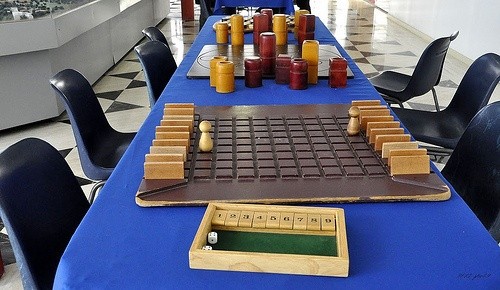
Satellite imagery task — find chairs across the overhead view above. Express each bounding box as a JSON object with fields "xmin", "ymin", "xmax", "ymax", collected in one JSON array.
[
  {"xmin": 440, "ymin": 101, "xmax": 500, "ymax": 244},
  {"xmin": 49, "ymin": 68, "xmax": 138, "ymax": 205},
  {"xmin": 195, "ymin": 0, "xmax": 311, "ymax": 32},
  {"xmin": 369, "ymin": 31, "xmax": 459, "ymax": 111},
  {"xmin": 390, "ymin": 53, "xmax": 500, "ymax": 163},
  {"xmin": 142, "ymin": 26, "xmax": 170, "ymax": 48},
  {"xmin": 0, "ymin": 137, "xmax": 91, "ymax": 290},
  {"xmin": 134, "ymin": 40, "xmax": 177, "ymax": 110}
]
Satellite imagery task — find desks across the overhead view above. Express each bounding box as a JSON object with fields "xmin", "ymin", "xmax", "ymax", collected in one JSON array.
[
  {"xmin": 53, "ymin": 16, "xmax": 500, "ymax": 290},
  {"xmin": 214, "ymin": 0, "xmax": 295, "ymax": 16}
]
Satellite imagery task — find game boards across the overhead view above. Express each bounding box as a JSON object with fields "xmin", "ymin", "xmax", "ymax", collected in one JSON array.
[
  {"xmin": 186, "ymin": 44, "xmax": 354, "ymax": 79},
  {"xmin": 135, "ymin": 104, "xmax": 451, "ymax": 207}
]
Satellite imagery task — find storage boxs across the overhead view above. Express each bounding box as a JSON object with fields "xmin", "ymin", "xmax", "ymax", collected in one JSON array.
[{"xmin": 188, "ymin": 201, "xmax": 349, "ymax": 278}]
